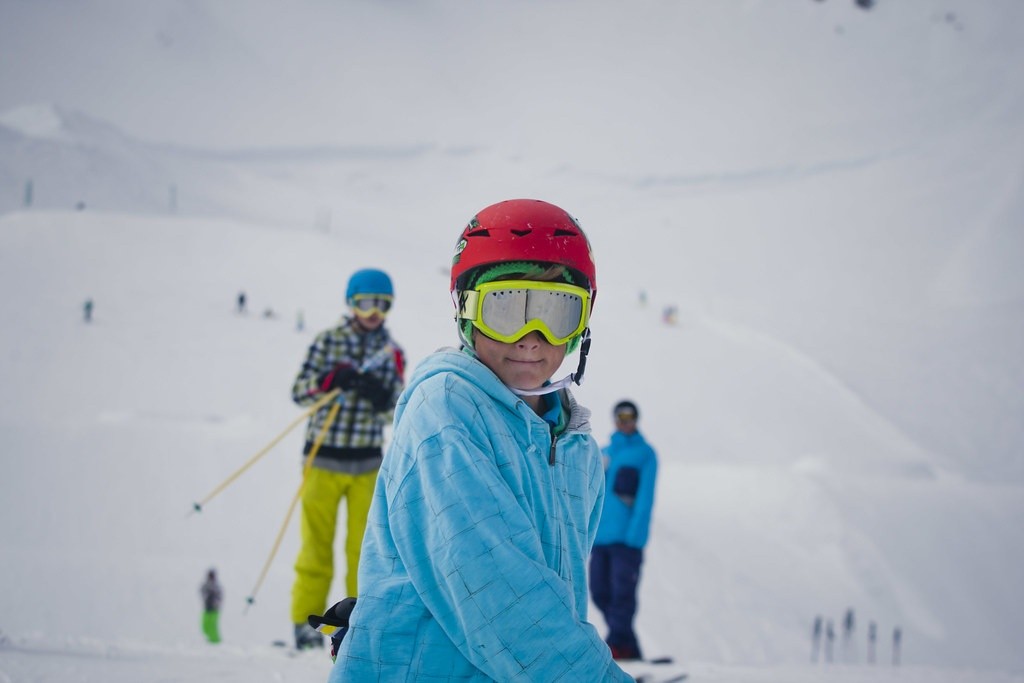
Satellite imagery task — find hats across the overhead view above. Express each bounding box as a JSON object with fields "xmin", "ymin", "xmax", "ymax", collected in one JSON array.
[
  {"xmin": 460, "ymin": 262, "xmax": 587, "ymax": 355},
  {"xmin": 614, "ymin": 401, "xmax": 637, "ymax": 419}
]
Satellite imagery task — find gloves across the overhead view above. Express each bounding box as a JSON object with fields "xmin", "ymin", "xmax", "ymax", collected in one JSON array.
[
  {"xmin": 356, "ymin": 373, "xmax": 391, "ymax": 414},
  {"xmin": 315, "ymin": 368, "xmax": 360, "ymax": 393}
]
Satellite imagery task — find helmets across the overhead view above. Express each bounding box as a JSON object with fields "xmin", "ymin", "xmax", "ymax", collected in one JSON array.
[
  {"xmin": 450, "ymin": 198, "xmax": 597, "ymax": 317},
  {"xmin": 346, "ymin": 269, "xmax": 395, "ymax": 305}
]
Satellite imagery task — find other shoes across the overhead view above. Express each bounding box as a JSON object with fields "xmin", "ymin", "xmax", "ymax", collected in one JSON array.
[
  {"xmin": 605, "ymin": 641, "xmax": 642, "ymax": 661},
  {"xmin": 296, "ymin": 622, "xmax": 324, "ymax": 649}
]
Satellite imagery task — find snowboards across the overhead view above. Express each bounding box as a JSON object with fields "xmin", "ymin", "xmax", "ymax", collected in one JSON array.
[{"xmin": 614, "ymin": 658, "xmax": 673, "ymax": 663}]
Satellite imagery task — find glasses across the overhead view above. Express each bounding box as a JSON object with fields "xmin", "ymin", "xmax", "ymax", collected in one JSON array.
[
  {"xmin": 352, "ymin": 292, "xmax": 393, "ymax": 320},
  {"xmin": 471, "ymin": 280, "xmax": 590, "ymax": 346}
]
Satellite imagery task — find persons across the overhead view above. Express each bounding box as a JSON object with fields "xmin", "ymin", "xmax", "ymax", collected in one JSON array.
[
  {"xmin": 200, "ymin": 570, "xmax": 221, "ymax": 642},
  {"xmin": 588, "ymin": 401, "xmax": 656, "ymax": 662},
  {"xmin": 292, "ymin": 269, "xmax": 404, "ymax": 648},
  {"xmin": 324, "ymin": 199, "xmax": 636, "ymax": 683}
]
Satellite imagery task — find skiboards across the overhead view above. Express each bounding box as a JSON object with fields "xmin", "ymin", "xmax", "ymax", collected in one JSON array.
[{"xmin": 296, "ymin": 638, "xmax": 324, "ymax": 650}]
